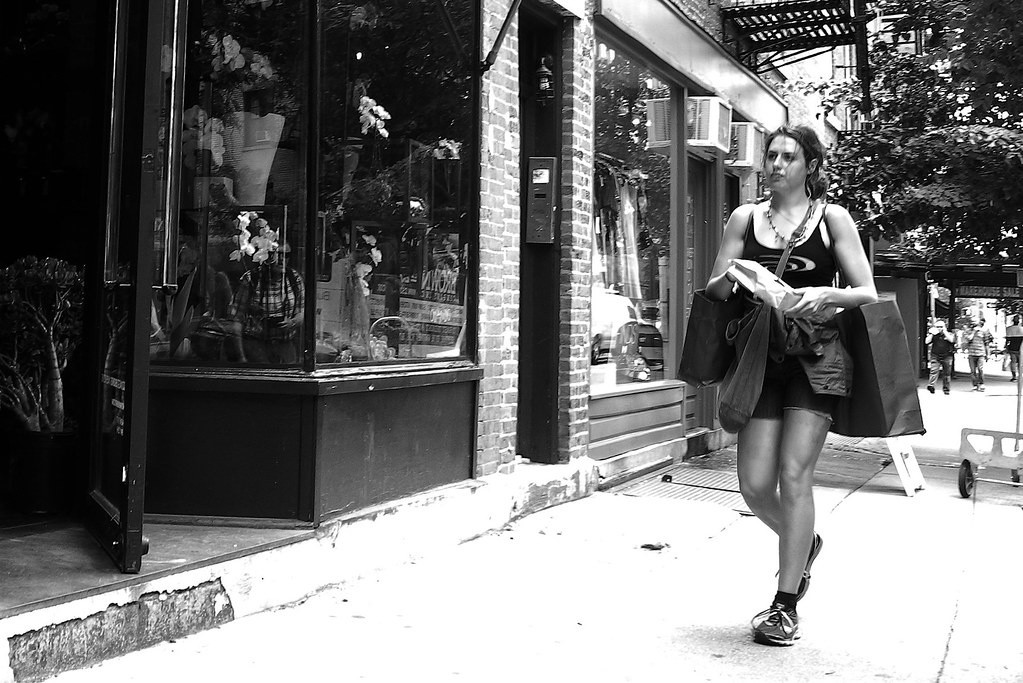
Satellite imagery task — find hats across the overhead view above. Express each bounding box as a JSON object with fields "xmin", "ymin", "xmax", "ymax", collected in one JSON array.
[{"xmin": 935, "ymin": 321, "xmax": 944, "ymax": 327}]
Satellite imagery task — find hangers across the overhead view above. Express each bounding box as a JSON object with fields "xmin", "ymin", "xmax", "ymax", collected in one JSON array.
[{"xmin": 595, "ymin": 152, "xmax": 641, "ymax": 188}]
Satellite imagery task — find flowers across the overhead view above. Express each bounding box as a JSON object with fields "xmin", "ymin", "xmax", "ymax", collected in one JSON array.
[
  {"xmin": 350, "ymin": 89, "xmax": 392, "ymax": 143},
  {"xmin": 197, "ymin": 29, "xmax": 296, "ymax": 117},
  {"xmin": 422, "ymin": 137, "xmax": 460, "ymax": 165},
  {"xmin": 230, "ymin": 210, "xmax": 282, "ymax": 271},
  {"xmin": 334, "ymin": 230, "xmax": 384, "ymax": 297}
]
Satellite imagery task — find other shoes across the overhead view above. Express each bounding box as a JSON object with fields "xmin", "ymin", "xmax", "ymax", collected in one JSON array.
[
  {"xmin": 927, "ymin": 385, "xmax": 934, "ymax": 394},
  {"xmin": 943, "ymin": 387, "xmax": 948, "ymax": 395},
  {"xmin": 1010, "ymin": 378, "xmax": 1017, "ymax": 382},
  {"xmin": 972, "ymin": 385, "xmax": 985, "ymax": 392}
]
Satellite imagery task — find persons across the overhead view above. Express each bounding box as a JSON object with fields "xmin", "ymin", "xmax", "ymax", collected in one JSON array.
[
  {"xmin": 1004, "ymin": 315, "xmax": 1023, "ymax": 382},
  {"xmin": 926, "ymin": 320, "xmax": 953, "ymax": 395},
  {"xmin": 705, "ymin": 124, "xmax": 879, "ymax": 646},
  {"xmin": 958, "ymin": 318, "xmax": 989, "ymax": 392}
]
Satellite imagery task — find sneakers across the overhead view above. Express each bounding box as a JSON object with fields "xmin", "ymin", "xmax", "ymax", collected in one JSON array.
[
  {"xmin": 751, "ymin": 601, "xmax": 801, "ymax": 646},
  {"xmin": 774, "ymin": 531, "xmax": 823, "ymax": 602}
]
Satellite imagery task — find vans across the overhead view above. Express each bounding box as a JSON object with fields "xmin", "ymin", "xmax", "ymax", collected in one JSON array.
[{"xmin": 592, "ymin": 287, "xmax": 646, "ymax": 364}]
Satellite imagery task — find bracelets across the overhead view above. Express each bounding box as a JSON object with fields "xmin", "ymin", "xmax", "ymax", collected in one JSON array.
[{"xmin": 725, "ymin": 273, "xmax": 735, "ymax": 282}]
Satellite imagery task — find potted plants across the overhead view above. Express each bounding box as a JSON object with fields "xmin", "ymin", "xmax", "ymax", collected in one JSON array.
[{"xmin": 0, "ymin": 254, "xmax": 91, "ymax": 513}]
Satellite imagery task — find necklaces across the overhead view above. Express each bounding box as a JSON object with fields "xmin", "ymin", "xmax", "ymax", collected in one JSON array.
[{"xmin": 767, "ymin": 200, "xmax": 813, "ymax": 245}]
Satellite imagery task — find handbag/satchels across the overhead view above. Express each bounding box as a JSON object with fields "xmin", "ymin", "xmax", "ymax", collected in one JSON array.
[
  {"xmin": 717, "ymin": 304, "xmax": 772, "ymax": 434},
  {"xmin": 676, "ymin": 290, "xmax": 755, "ymax": 388},
  {"xmin": 1002, "ymin": 353, "xmax": 1011, "ymax": 372},
  {"xmin": 828, "ymin": 298, "xmax": 925, "ymax": 437}
]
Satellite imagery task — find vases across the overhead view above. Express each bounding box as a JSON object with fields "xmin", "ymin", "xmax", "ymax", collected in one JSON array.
[{"xmin": 226, "ymin": 113, "xmax": 286, "ymax": 209}]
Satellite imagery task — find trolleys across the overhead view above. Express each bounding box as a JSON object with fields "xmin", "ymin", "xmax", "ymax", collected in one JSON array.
[{"xmin": 958, "ymin": 428, "xmax": 1023, "ymax": 499}]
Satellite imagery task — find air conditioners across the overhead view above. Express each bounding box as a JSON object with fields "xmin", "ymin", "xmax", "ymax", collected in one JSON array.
[
  {"xmin": 687, "ymin": 97, "xmax": 733, "ymax": 152},
  {"xmin": 646, "ymin": 98, "xmax": 672, "ymax": 154},
  {"xmin": 723, "ymin": 122, "xmax": 767, "ymax": 173}
]
